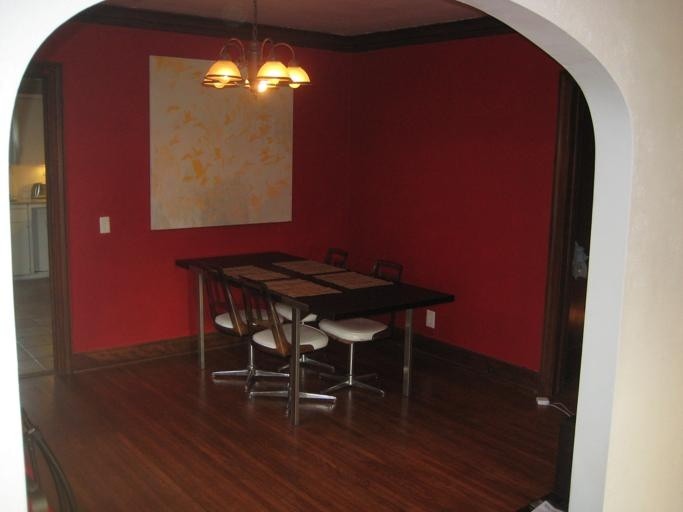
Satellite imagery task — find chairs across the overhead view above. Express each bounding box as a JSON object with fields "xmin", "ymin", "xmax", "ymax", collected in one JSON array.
[{"xmin": 200, "ymin": 248, "xmax": 404, "ymax": 416}]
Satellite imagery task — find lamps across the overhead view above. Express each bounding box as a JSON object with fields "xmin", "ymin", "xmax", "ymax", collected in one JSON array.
[{"xmin": 202, "ymin": 2, "xmax": 315, "ymax": 93}]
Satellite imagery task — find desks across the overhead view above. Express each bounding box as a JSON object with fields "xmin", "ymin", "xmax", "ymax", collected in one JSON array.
[{"xmin": 176, "ymin": 255, "xmax": 455, "ymax": 436}]
